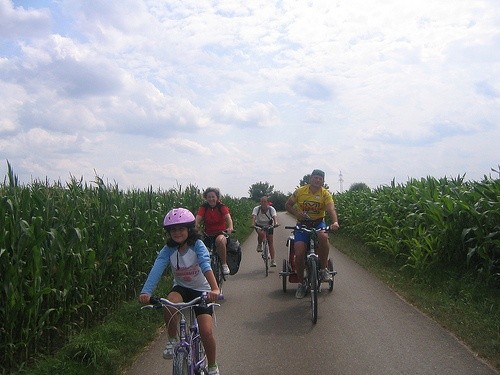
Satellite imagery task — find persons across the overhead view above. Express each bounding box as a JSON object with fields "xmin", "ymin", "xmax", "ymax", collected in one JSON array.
[
  {"xmin": 195, "ymin": 187, "xmax": 233, "ymax": 275},
  {"xmin": 285, "ymin": 170, "xmax": 339, "ymax": 298},
  {"xmin": 138, "ymin": 208, "xmax": 221, "ymax": 375},
  {"xmin": 252, "ymin": 196, "xmax": 278, "ymax": 266}
]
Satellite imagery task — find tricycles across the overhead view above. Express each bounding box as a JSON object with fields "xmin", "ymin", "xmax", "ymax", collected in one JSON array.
[{"xmin": 279, "ymin": 225, "xmax": 338, "ymax": 324}]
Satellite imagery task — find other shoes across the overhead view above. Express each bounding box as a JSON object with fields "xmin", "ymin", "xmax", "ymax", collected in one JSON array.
[
  {"xmin": 208, "ymin": 364, "xmax": 219, "ymax": 375},
  {"xmin": 322, "ymin": 269, "xmax": 332, "ymax": 282},
  {"xmin": 271, "ymin": 261, "xmax": 276, "ymax": 267},
  {"xmin": 222, "ymin": 265, "xmax": 230, "ymax": 275},
  {"xmin": 257, "ymin": 244, "xmax": 263, "ymax": 252},
  {"xmin": 296, "ymin": 283, "xmax": 306, "ymax": 298},
  {"xmin": 163, "ymin": 339, "xmax": 179, "ymax": 360}
]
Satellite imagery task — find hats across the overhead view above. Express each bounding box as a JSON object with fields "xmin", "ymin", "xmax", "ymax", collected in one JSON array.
[{"xmin": 312, "ymin": 170, "xmax": 324, "ymax": 177}]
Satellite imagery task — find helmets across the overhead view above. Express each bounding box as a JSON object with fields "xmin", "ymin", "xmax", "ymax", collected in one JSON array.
[{"xmin": 163, "ymin": 208, "xmax": 195, "ymax": 226}]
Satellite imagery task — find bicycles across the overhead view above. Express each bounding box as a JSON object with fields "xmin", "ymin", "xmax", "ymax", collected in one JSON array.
[
  {"xmin": 192, "ymin": 227, "xmax": 237, "ymax": 297},
  {"xmin": 140, "ymin": 292, "xmax": 224, "ymax": 375},
  {"xmin": 250, "ymin": 224, "xmax": 280, "ymax": 277}
]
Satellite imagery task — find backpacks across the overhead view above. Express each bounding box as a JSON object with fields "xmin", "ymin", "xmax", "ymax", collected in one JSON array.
[{"xmin": 226, "ymin": 237, "xmax": 242, "ymax": 275}]
